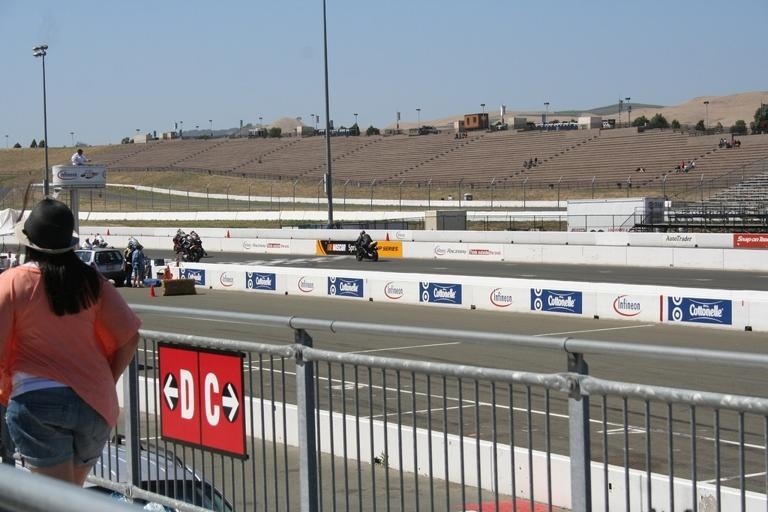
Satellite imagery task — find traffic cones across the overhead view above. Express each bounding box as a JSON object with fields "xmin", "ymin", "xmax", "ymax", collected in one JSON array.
[
  {"xmin": 149, "ymin": 284, "xmax": 156, "ymax": 298},
  {"xmin": 225, "ymin": 231, "xmax": 231, "ymax": 238},
  {"xmin": 107, "ymin": 229, "xmax": 111, "ymax": 235}
]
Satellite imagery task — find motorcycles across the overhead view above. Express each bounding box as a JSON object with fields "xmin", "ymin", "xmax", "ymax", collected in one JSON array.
[
  {"xmin": 354, "ymin": 240, "xmax": 379, "ymax": 262},
  {"xmin": 172, "ymin": 234, "xmax": 204, "ymax": 262}
]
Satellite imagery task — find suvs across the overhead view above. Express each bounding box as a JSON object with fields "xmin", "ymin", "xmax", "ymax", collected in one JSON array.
[
  {"xmin": 81, "ymin": 431, "xmax": 236, "ymax": 512},
  {"xmin": 75, "ymin": 248, "xmax": 127, "ymax": 285}
]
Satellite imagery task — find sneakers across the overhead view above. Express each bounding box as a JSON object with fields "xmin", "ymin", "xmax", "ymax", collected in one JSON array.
[
  {"xmin": 133, "ymin": 285, "xmax": 143, "ymax": 288},
  {"xmin": 125, "ymin": 283, "xmax": 132, "ymax": 287}
]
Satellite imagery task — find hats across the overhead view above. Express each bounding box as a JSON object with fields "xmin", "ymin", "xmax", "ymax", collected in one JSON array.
[{"xmin": 14, "ymin": 197, "xmax": 80, "ymax": 254}]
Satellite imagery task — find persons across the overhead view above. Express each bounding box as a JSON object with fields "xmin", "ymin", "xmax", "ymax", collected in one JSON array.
[
  {"xmin": 128, "ymin": 235, "xmax": 139, "ymax": 247},
  {"xmin": 83, "ymin": 237, "xmax": 92, "ymax": 250},
  {"xmin": 123, "ymin": 244, "xmax": 134, "ymax": 287},
  {"xmin": 356, "ymin": 231, "xmax": 372, "ymax": 255},
  {"xmin": 718, "ymin": 136, "xmax": 741, "ymax": 148},
  {"xmin": 679, "ymin": 159, "xmax": 684, "ymax": 170},
  {"xmin": 675, "ymin": 166, "xmax": 680, "ymax": 173},
  {"xmin": 95, "ymin": 232, "xmax": 104, "ymax": 243},
  {"xmin": 131, "ymin": 244, "xmax": 145, "ymax": 288},
  {"xmin": 0, "ymin": 199, "xmax": 141, "ymax": 486}
]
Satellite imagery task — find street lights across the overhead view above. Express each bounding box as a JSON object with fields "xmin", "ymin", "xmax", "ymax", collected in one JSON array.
[
  {"xmin": 480, "ymin": 103, "xmax": 485, "ymax": 117},
  {"xmin": 32, "ymin": 44, "xmax": 49, "ymax": 197},
  {"xmin": 544, "ymin": 103, "xmax": 549, "ymax": 123},
  {"xmin": 416, "ymin": 108, "xmax": 420, "ymax": 129},
  {"xmin": 703, "ymin": 100, "xmax": 709, "ymax": 127},
  {"xmin": 625, "ymin": 97, "xmax": 631, "ymax": 127},
  {"xmin": 354, "ymin": 113, "xmax": 358, "ymax": 125}
]
